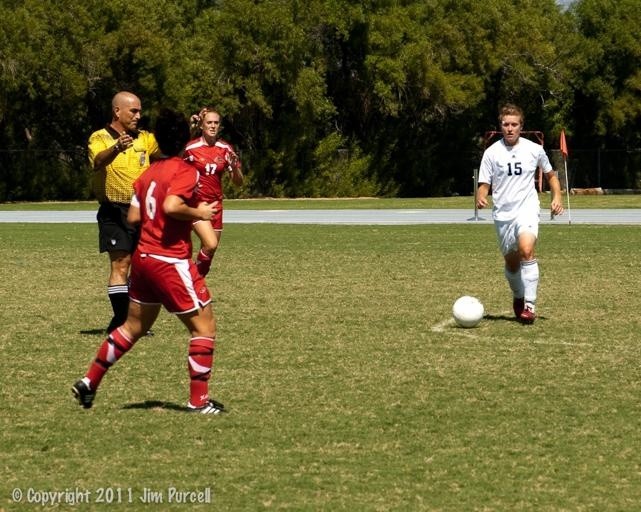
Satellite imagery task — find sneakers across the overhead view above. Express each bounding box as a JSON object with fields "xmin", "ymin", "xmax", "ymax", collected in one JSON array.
[
  {"xmin": 186, "ymin": 401, "xmax": 227, "ymax": 414},
  {"xmin": 70, "ymin": 376, "xmax": 94, "ymax": 408},
  {"xmin": 512, "ymin": 300, "xmax": 536, "ymax": 324}
]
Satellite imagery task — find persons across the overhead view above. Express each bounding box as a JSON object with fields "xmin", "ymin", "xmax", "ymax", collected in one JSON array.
[
  {"xmin": 71, "ymin": 92, "xmax": 243, "ymax": 414},
  {"xmin": 475, "ymin": 105, "xmax": 564, "ymax": 324}
]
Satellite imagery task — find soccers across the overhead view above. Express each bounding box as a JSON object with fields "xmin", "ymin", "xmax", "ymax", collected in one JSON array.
[{"xmin": 452, "ymin": 296, "xmax": 484, "ymax": 327}]
